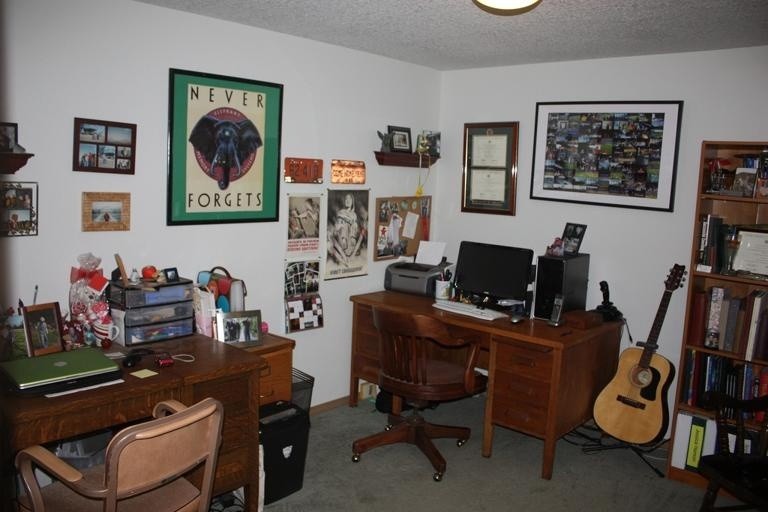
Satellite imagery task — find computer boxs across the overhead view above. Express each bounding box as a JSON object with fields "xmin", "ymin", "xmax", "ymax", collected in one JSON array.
[{"xmin": 534, "ymin": 253, "xmax": 590, "ymax": 320}]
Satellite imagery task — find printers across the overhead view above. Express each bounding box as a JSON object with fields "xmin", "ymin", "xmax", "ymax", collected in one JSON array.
[{"xmin": 383, "ymin": 257, "xmax": 456, "ymax": 294}]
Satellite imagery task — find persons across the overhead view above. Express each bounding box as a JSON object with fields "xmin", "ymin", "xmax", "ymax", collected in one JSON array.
[
  {"xmin": 334, "ymin": 194, "xmax": 359, "ymax": 259},
  {"xmin": 103, "ymin": 210, "xmax": 113, "ymax": 223},
  {"xmin": 80, "ymin": 146, "xmax": 133, "ymax": 168},
  {"xmin": 17, "ymin": 192, "xmax": 31, "ymax": 208},
  {"xmin": 292, "ymin": 199, "xmax": 320, "ymax": 230},
  {"xmin": 225, "ymin": 316, "xmax": 257, "ymax": 341},
  {"xmin": 35, "ymin": 316, "xmax": 51, "ymax": 349}
]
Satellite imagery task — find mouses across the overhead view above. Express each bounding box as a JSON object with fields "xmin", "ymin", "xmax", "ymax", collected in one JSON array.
[
  {"xmin": 123, "ymin": 356, "xmax": 136, "ymax": 367},
  {"xmin": 511, "ymin": 315, "xmax": 525, "ymax": 323}
]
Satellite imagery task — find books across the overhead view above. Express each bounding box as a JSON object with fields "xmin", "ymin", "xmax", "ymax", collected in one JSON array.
[{"xmin": 671, "ymin": 211, "xmax": 768, "ymax": 476}]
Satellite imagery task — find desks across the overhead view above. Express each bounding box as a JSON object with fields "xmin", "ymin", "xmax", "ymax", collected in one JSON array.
[
  {"xmin": 349, "ymin": 276, "xmax": 627, "ymax": 482},
  {"xmin": 1, "ymin": 322, "xmax": 267, "ymax": 511}
]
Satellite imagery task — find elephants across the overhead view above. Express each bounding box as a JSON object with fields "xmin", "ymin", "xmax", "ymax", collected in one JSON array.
[{"xmin": 188, "ymin": 114, "xmax": 263, "ymax": 190}]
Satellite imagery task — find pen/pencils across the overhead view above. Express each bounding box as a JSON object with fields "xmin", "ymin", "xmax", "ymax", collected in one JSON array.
[
  {"xmin": 438, "ymin": 269, "xmax": 452, "ymax": 281},
  {"xmin": 33, "ymin": 284, "xmax": 38, "ymax": 305}
]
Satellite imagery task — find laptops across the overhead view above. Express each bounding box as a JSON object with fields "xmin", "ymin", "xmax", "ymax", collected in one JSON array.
[{"xmin": 1, "ymin": 345, "xmax": 120, "ymax": 390}]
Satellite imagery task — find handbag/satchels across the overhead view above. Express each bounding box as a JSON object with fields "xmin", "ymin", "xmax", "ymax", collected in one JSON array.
[
  {"xmin": 197, "ymin": 266, "xmax": 246, "ymax": 312},
  {"xmin": 193, "ymin": 282, "xmax": 216, "ymax": 339}
]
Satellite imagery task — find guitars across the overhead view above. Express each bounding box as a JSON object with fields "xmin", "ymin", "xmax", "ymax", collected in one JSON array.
[{"xmin": 593, "ymin": 264, "xmax": 687, "ymax": 447}]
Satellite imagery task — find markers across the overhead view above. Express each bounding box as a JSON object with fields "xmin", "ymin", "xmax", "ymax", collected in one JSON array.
[{"xmin": 18, "ymin": 298, "xmax": 24, "ymax": 315}]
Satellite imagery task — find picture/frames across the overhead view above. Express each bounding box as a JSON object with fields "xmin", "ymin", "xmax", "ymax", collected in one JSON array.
[
  {"xmin": 19, "ymin": 301, "xmax": 65, "ymax": 357},
  {"xmin": 1, "ymin": 180, "xmax": 39, "ymax": 238},
  {"xmin": 529, "ymin": 99, "xmax": 685, "ymax": 213},
  {"xmin": 72, "ymin": 117, "xmax": 137, "ymax": 175},
  {"xmin": 387, "ymin": 124, "xmax": 414, "ymax": 154},
  {"xmin": 166, "ymin": 66, "xmax": 285, "ymax": 227},
  {"xmin": 718, "ymin": 224, "xmax": 768, "ymax": 280},
  {"xmin": 560, "ymin": 222, "xmax": 588, "ymax": 257},
  {"xmin": 83, "ymin": 191, "xmax": 131, "ymax": 232},
  {"xmin": 460, "ymin": 121, "xmax": 519, "ymax": 216},
  {"xmin": 217, "ymin": 308, "xmax": 264, "ymax": 350}
]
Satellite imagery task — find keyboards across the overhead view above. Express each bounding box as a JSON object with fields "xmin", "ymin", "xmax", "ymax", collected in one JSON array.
[{"xmin": 432, "ymin": 300, "xmax": 509, "ymax": 321}]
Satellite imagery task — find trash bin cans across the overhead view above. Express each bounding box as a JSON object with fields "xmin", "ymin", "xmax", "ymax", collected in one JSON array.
[{"xmin": 292, "ymin": 367, "xmax": 315, "ymax": 412}]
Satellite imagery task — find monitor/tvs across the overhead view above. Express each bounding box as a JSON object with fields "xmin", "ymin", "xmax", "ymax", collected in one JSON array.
[{"xmin": 453, "ymin": 240, "xmax": 534, "ymax": 309}]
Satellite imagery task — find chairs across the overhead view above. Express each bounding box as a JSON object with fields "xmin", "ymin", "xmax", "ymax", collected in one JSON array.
[
  {"xmin": 691, "ymin": 386, "xmax": 768, "ymax": 512},
  {"xmin": 13, "ymin": 396, "xmax": 222, "ymax": 511},
  {"xmin": 351, "ymin": 305, "xmax": 485, "ymax": 481}
]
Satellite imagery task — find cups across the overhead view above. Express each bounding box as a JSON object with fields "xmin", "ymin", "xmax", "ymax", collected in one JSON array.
[{"xmin": 435, "ymin": 279, "xmax": 451, "ymax": 299}]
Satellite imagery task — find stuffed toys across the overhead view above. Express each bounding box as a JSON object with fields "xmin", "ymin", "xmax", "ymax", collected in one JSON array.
[{"xmin": 75, "ymin": 285, "xmax": 98, "ymax": 305}]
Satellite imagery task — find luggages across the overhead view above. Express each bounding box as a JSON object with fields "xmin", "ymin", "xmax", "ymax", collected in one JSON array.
[{"xmin": 259, "ymin": 401, "xmax": 310, "ymax": 505}]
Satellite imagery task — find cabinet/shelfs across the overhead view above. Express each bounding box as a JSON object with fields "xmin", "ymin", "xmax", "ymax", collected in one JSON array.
[
  {"xmin": 106, "ymin": 275, "xmax": 195, "ymax": 343},
  {"xmin": 662, "ymin": 140, "xmax": 768, "ymax": 483},
  {"xmin": 228, "ymin": 328, "xmax": 297, "ymax": 409}
]
